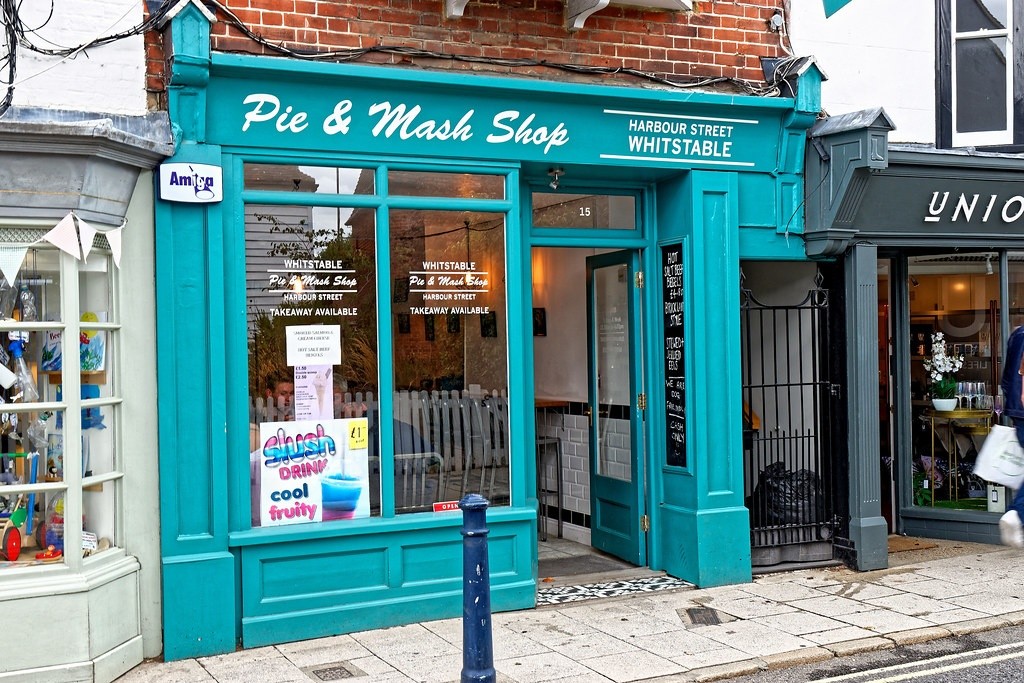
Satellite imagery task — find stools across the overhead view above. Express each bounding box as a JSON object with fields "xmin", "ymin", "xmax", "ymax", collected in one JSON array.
[{"xmin": 421, "ymin": 393, "xmax": 563, "ymax": 540}]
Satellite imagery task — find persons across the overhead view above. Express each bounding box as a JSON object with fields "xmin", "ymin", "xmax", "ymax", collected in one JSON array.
[
  {"xmin": 251, "ymin": 370, "xmax": 294, "ymax": 421},
  {"xmin": 333, "ymin": 377, "xmax": 427, "ymax": 511},
  {"xmin": 997, "ymin": 324, "xmax": 1024, "ymax": 548}
]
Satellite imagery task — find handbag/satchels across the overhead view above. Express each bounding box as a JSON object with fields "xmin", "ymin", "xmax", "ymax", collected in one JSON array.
[{"xmin": 972, "ymin": 424, "xmax": 1024, "ymax": 490}]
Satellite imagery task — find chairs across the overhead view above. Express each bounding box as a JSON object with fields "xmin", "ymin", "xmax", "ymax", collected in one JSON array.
[{"xmin": 369, "ymin": 451, "xmax": 445, "ymax": 516}]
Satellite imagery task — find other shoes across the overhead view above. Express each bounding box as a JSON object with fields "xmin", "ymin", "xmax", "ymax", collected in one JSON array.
[{"xmin": 999, "ymin": 510, "xmax": 1024, "ymax": 548}]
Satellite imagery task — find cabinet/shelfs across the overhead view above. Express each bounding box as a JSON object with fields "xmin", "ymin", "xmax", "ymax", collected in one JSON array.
[{"xmin": 36, "ymin": 370, "xmax": 107, "ymax": 492}]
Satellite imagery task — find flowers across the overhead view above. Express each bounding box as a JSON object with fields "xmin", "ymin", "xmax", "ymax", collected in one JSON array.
[{"xmin": 923, "ymin": 332, "xmax": 966, "ymax": 399}]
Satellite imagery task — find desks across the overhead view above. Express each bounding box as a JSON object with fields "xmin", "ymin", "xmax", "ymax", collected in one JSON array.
[{"xmin": 924, "ymin": 407, "xmax": 994, "ymax": 508}]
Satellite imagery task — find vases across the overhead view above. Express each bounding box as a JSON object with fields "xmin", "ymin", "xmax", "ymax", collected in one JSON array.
[{"xmin": 932, "ymin": 399, "xmax": 957, "ymax": 411}]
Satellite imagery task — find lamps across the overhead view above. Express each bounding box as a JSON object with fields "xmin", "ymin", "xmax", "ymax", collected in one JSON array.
[
  {"xmin": 910, "ymin": 275, "xmax": 920, "ymax": 288},
  {"xmin": 455, "ymin": 221, "xmax": 483, "ymax": 295},
  {"xmin": 985, "ymin": 253, "xmax": 994, "ymax": 275}
]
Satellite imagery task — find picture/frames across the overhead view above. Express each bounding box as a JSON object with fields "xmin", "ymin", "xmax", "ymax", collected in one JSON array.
[
  {"xmin": 479, "ymin": 311, "xmax": 497, "ymax": 337},
  {"xmin": 398, "ymin": 313, "xmax": 411, "ymax": 334},
  {"xmin": 424, "ymin": 310, "xmax": 435, "ymax": 341},
  {"xmin": 447, "ymin": 309, "xmax": 460, "ymax": 333},
  {"xmin": 393, "ymin": 277, "xmax": 410, "ymax": 303},
  {"xmin": 533, "ymin": 307, "xmax": 547, "ymax": 336}
]
